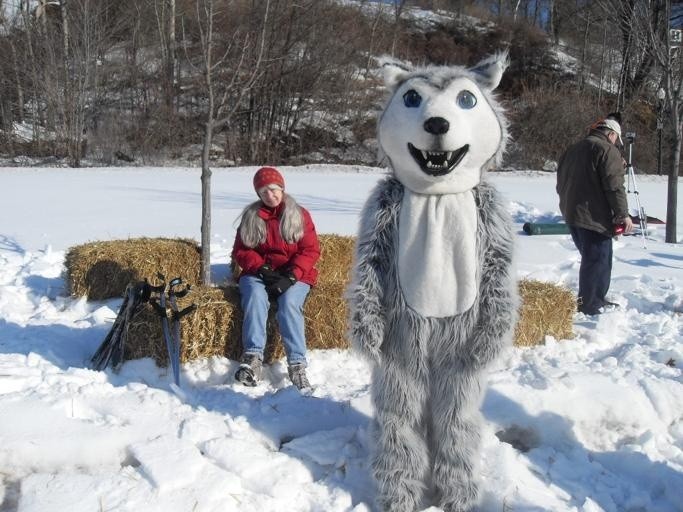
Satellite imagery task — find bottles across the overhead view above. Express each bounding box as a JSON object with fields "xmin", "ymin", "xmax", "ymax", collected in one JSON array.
[{"xmin": 612, "ymin": 222, "xmax": 625, "ymax": 236}]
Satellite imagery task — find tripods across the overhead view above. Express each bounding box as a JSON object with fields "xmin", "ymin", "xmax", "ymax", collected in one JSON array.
[{"xmin": 626, "ymin": 144, "xmax": 649, "ymax": 249}]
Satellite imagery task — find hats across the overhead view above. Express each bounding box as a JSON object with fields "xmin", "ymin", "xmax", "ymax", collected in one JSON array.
[
  {"xmin": 595, "ymin": 119, "xmax": 624, "ymax": 146},
  {"xmin": 253, "ymin": 167, "xmax": 285, "ymax": 190}
]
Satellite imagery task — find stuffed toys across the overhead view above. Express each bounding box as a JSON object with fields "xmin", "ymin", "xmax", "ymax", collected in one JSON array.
[{"xmin": 343, "ymin": 47, "xmax": 520, "ymax": 510}]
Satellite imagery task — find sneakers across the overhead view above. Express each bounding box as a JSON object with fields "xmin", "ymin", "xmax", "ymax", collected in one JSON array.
[
  {"xmin": 288, "ymin": 364, "xmax": 311, "ymax": 396},
  {"xmin": 594, "ymin": 301, "xmax": 620, "ymax": 314},
  {"xmin": 234, "ymin": 352, "xmax": 265, "ymax": 387}
]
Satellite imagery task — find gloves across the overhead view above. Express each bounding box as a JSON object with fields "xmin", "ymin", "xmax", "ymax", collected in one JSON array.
[
  {"xmin": 259, "ymin": 266, "xmax": 295, "ymax": 299},
  {"xmin": 621, "ymin": 217, "xmax": 633, "ymax": 233}
]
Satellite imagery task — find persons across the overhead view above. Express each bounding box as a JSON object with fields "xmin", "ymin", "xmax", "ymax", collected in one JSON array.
[
  {"xmin": 555, "ymin": 117, "xmax": 634, "ymax": 316},
  {"xmin": 229, "ymin": 165, "xmax": 319, "ymax": 394}
]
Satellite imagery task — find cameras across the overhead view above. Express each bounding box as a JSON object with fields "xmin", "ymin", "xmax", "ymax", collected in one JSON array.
[{"xmin": 625, "ymin": 132, "xmax": 636, "ymax": 143}]
